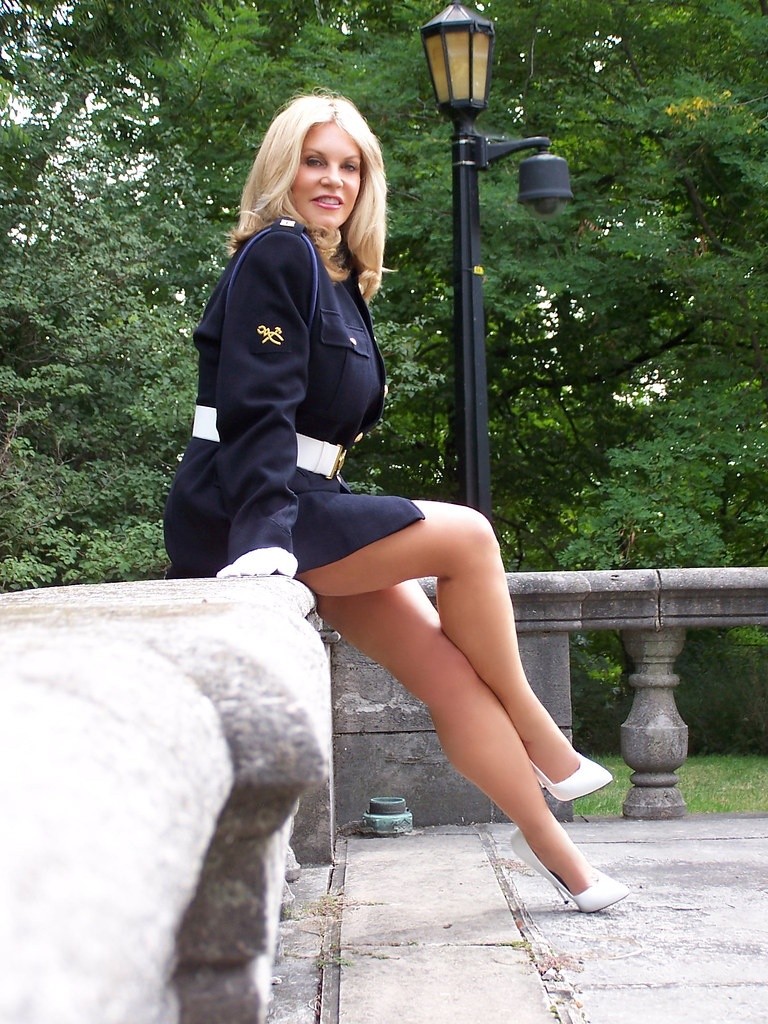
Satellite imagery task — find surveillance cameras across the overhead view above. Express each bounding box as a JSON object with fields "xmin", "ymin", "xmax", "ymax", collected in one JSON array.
[{"xmin": 518, "ymin": 155, "xmax": 574, "ymax": 220}]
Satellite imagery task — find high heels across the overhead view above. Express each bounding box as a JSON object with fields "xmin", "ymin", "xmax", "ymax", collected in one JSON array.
[
  {"xmin": 528, "ymin": 744, "xmax": 613, "ymax": 801},
  {"xmin": 511, "ymin": 827, "xmax": 630, "ymax": 911}
]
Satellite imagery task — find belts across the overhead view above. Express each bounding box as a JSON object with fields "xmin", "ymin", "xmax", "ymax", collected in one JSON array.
[{"xmin": 192, "ymin": 396, "xmax": 349, "ymax": 481}]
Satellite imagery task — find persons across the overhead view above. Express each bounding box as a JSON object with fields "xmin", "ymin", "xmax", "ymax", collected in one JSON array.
[{"xmin": 164, "ymin": 95, "xmax": 630, "ymax": 913}]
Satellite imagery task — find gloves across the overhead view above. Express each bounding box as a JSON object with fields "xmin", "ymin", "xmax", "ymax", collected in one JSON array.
[{"xmin": 217, "ymin": 542, "xmax": 300, "ymax": 579}]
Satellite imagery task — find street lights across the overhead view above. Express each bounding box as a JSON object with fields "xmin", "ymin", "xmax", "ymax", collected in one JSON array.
[{"xmin": 417, "ymin": 0, "xmax": 576, "ymax": 526}]
